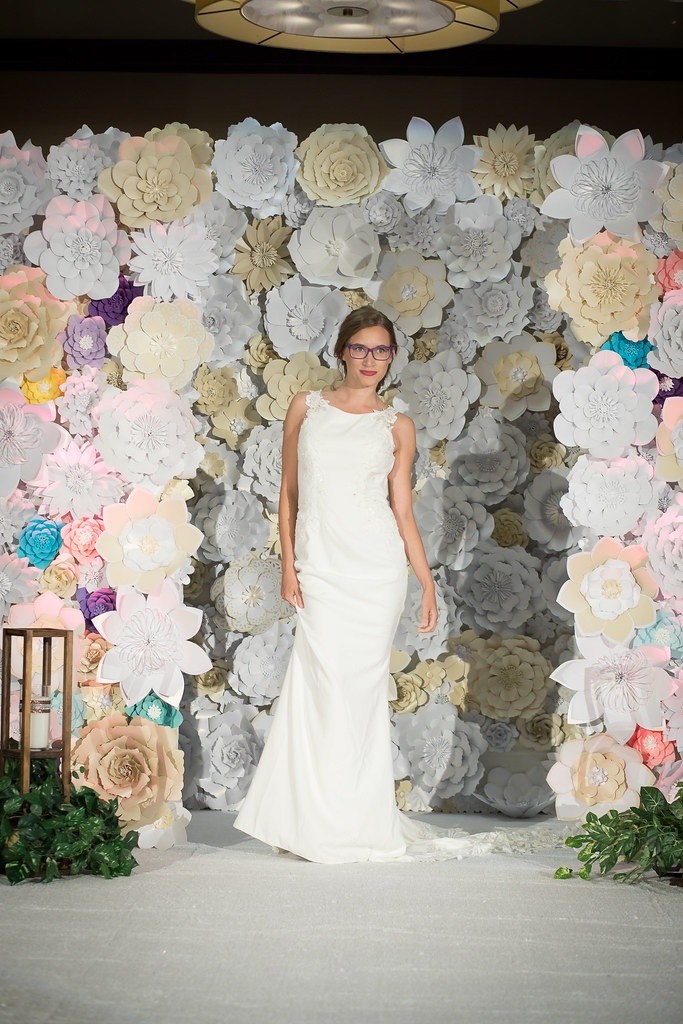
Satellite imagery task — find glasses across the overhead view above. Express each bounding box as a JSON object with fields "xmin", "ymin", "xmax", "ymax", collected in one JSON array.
[{"xmin": 346, "ymin": 342, "xmax": 393, "ymax": 360}]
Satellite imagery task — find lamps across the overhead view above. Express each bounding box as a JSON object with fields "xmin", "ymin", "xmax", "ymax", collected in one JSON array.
[{"xmin": 194, "ymin": 0, "xmax": 537, "ymax": 55}]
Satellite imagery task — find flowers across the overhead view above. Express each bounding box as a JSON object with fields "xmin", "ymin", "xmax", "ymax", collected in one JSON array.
[{"xmin": 0, "ymin": 110, "xmax": 683, "ymax": 826}]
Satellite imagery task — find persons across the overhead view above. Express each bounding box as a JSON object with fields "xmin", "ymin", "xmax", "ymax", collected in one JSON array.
[{"xmin": 232, "ymin": 305, "xmax": 509, "ymax": 864}]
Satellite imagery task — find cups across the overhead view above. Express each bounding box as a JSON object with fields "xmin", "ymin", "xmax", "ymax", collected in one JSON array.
[{"xmin": 18, "ymin": 696, "xmax": 51, "ymax": 751}]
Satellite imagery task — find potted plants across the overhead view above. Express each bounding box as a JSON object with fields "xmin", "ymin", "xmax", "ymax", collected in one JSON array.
[
  {"xmin": 0, "ymin": 734, "xmax": 140, "ymax": 888},
  {"xmin": 551, "ymin": 777, "xmax": 683, "ymax": 885}
]
